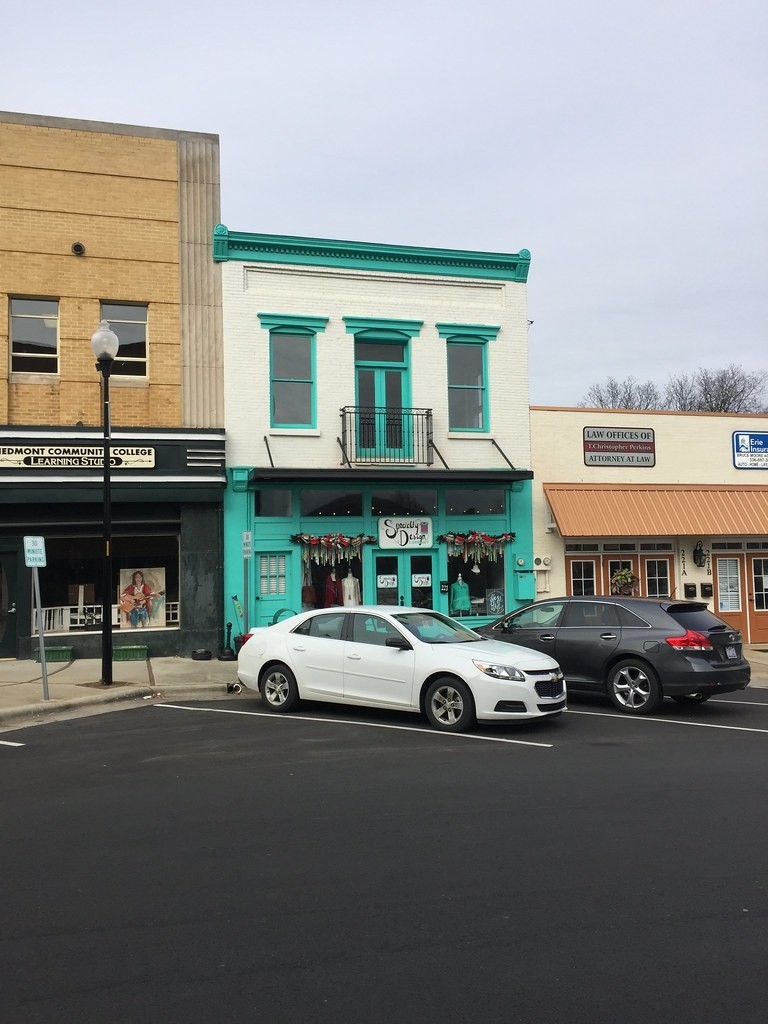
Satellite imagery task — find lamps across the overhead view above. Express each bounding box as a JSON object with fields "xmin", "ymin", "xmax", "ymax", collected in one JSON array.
[{"xmin": 693, "ymin": 541, "xmax": 707, "ymax": 567}]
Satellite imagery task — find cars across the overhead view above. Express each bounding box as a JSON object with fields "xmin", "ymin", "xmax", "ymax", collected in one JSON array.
[
  {"xmin": 237, "ymin": 605, "xmax": 569, "ymax": 732},
  {"xmin": 449, "ymin": 595, "xmax": 752, "ymax": 716}
]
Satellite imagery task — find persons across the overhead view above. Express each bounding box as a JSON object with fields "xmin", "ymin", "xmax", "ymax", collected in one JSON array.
[
  {"xmin": 450, "ymin": 573, "xmax": 472, "ymax": 616},
  {"xmin": 341, "ymin": 570, "xmax": 362, "ymax": 606},
  {"xmin": 324, "ymin": 570, "xmax": 343, "ymax": 610}
]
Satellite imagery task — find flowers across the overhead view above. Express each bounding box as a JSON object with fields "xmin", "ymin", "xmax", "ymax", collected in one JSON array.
[{"xmin": 611, "ymin": 568, "xmax": 639, "ymax": 595}]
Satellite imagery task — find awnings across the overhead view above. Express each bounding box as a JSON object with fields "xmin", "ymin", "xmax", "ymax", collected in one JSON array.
[{"xmin": 544, "ymin": 481, "xmax": 767, "ymax": 540}]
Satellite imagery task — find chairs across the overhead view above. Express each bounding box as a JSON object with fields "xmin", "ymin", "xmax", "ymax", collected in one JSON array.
[
  {"xmin": 338, "ymin": 618, "xmax": 366, "ymax": 643},
  {"xmin": 603, "ymin": 607, "xmax": 630, "ymax": 626},
  {"xmin": 563, "ymin": 606, "xmax": 588, "ymax": 627},
  {"xmin": 385, "ymin": 621, "xmax": 396, "ymax": 639}
]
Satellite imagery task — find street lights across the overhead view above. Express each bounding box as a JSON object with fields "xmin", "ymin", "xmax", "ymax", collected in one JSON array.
[{"xmin": 91, "ymin": 318, "xmax": 121, "ymax": 685}]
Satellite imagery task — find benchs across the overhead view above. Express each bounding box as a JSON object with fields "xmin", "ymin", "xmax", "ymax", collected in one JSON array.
[
  {"xmin": 112, "ymin": 645, "xmax": 148, "ymax": 661},
  {"xmin": 308, "ymin": 623, "xmax": 337, "ymax": 639},
  {"xmin": 33, "ymin": 646, "xmax": 74, "ymax": 663}
]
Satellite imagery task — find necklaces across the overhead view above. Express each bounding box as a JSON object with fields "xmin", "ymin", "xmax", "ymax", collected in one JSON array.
[{"xmin": 346, "ymin": 577, "xmax": 354, "ymax": 600}]
